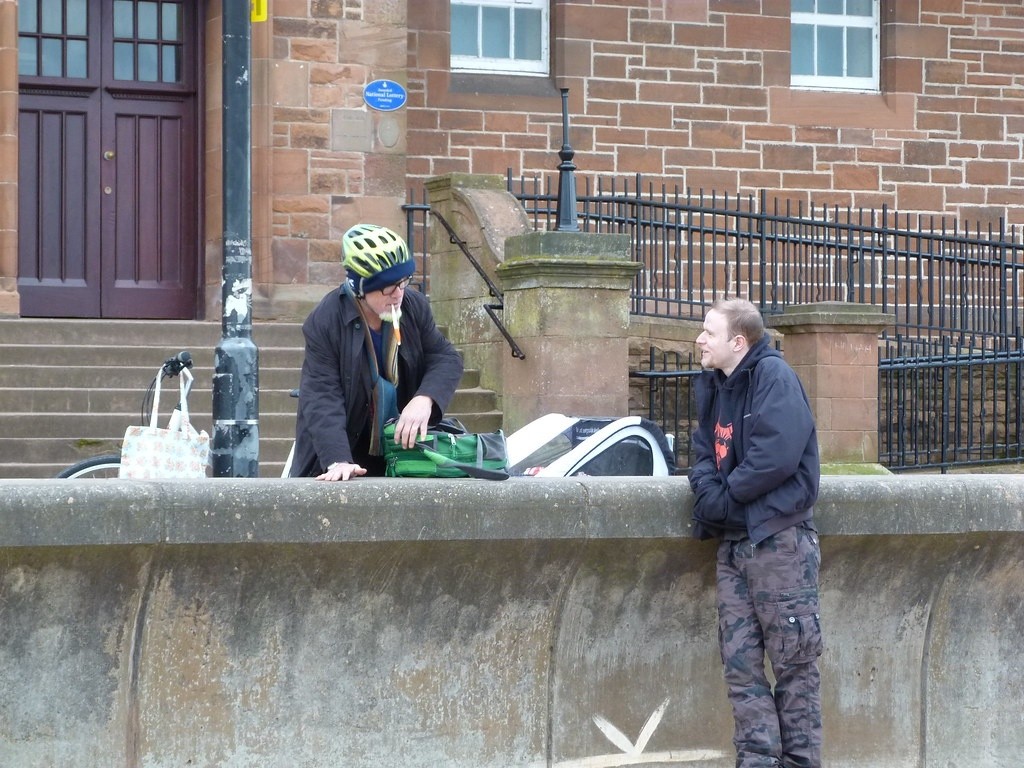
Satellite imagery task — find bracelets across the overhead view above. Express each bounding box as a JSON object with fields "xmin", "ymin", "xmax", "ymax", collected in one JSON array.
[{"xmin": 327, "ymin": 462, "xmax": 337, "ymax": 469}]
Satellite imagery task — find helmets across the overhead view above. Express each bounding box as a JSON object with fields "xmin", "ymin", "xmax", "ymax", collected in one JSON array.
[{"xmin": 342, "ymin": 224, "xmax": 414, "ymax": 293}]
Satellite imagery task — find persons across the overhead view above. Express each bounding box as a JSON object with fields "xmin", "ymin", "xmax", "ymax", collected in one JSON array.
[
  {"xmin": 286, "ymin": 223, "xmax": 463, "ymax": 481},
  {"xmin": 687, "ymin": 299, "xmax": 824, "ymax": 768}
]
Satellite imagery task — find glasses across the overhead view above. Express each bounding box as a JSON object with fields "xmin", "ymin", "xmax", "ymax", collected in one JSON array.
[{"xmin": 379, "ymin": 275, "xmax": 413, "ymax": 295}]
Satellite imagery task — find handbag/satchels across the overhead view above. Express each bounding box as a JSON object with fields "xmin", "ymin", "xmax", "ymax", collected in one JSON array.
[
  {"xmin": 383, "ymin": 417, "xmax": 509, "ymax": 481},
  {"xmin": 118, "ymin": 364, "xmax": 210, "ymax": 479}
]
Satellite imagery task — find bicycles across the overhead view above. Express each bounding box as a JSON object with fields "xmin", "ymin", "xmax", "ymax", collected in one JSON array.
[{"xmin": 57, "ymin": 351, "xmax": 299, "ymax": 478}]
